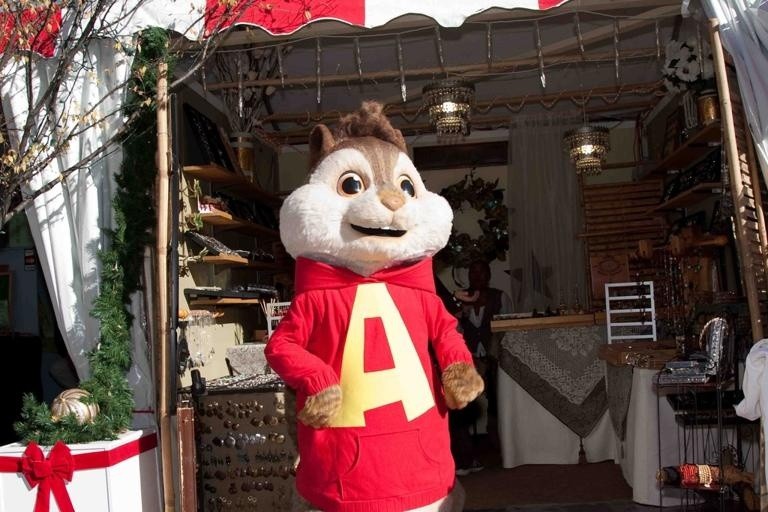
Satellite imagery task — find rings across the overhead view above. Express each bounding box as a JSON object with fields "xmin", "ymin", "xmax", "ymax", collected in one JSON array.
[{"xmin": 259, "ymin": 298, "xmax": 275, "ymax": 317}]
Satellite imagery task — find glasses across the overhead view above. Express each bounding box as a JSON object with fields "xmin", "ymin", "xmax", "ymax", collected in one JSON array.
[
  {"xmin": 563, "ymin": 65, "xmax": 610, "ymax": 175},
  {"xmin": 421, "ymin": 70, "xmax": 475, "ymax": 138}
]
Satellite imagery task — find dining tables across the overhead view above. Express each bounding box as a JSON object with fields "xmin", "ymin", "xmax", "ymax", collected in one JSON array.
[{"xmin": 213, "ymin": 42, "xmax": 293, "ymax": 181}]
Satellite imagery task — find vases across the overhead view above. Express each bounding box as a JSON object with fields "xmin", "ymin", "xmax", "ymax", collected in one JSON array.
[
  {"xmin": 662, "ymin": 37, "xmax": 714, "ymax": 95},
  {"xmin": 439, "ymin": 177, "xmax": 509, "ymax": 268}
]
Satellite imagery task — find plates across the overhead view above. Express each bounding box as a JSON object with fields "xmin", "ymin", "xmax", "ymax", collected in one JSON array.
[
  {"xmin": 637, "ymin": 60, "xmax": 768, "ymax": 352},
  {"xmin": 181, "ymin": 165, "xmax": 286, "ymax": 307},
  {"xmin": 0, "ymin": 427, "xmax": 165, "ymax": 512},
  {"xmin": 602, "ymin": 341, "xmax": 740, "ymax": 505},
  {"xmin": 657, "ymin": 313, "xmax": 754, "ymax": 511}
]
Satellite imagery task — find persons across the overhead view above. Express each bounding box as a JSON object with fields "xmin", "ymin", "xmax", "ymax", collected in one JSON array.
[
  {"xmin": 427, "ymin": 269, "xmax": 485, "ymax": 477},
  {"xmin": 458, "ymin": 259, "xmax": 516, "ymax": 394}
]
